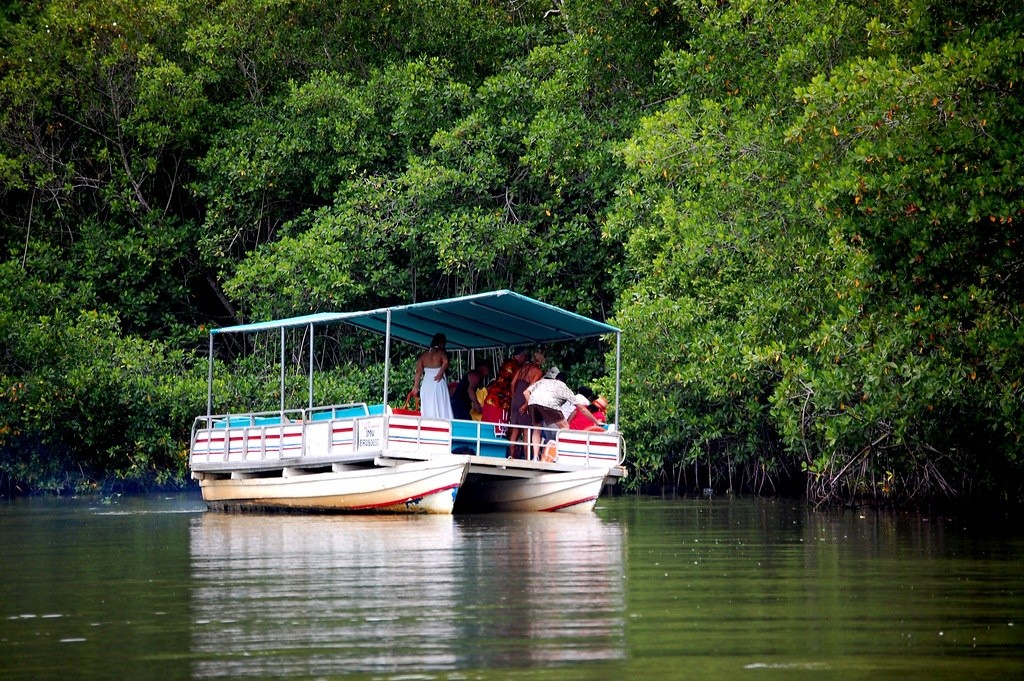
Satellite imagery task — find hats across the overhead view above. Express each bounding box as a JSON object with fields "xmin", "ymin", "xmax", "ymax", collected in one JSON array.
[
  {"xmin": 544, "ymin": 367, "xmax": 560, "ymax": 379},
  {"xmin": 593, "ymin": 397, "xmax": 608, "ymax": 410}
]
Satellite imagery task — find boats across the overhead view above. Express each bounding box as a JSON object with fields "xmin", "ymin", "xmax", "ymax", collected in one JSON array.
[{"xmin": 189, "ymin": 291, "xmax": 628, "ymax": 514}]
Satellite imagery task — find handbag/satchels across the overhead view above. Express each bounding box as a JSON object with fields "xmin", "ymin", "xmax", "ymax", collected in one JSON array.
[{"xmin": 389, "ymin": 392, "xmax": 421, "ymax": 429}]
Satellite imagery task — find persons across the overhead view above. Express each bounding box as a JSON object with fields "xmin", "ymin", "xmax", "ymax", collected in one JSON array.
[
  {"xmin": 411, "ymin": 333, "xmax": 454, "ymax": 418},
  {"xmin": 458, "ymin": 348, "xmax": 609, "ymax": 460}
]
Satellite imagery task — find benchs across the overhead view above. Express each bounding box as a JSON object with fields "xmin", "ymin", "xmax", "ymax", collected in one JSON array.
[
  {"xmin": 312, "ymin": 404, "xmax": 393, "ymax": 420},
  {"xmin": 451, "ymin": 422, "xmax": 508, "ymax": 457},
  {"xmin": 215, "ymin": 416, "xmax": 281, "ymax": 428}
]
